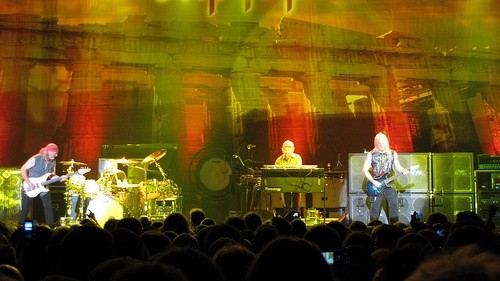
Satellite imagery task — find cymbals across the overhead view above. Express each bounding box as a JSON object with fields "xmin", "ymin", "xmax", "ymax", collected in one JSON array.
[
  {"xmin": 58, "ymin": 161, "xmax": 86, "ymax": 165},
  {"xmin": 141, "ymin": 149, "xmax": 167, "ymax": 165},
  {"xmin": 108, "ymin": 159, "xmax": 139, "ymax": 164},
  {"xmin": 113, "ymin": 183, "xmax": 139, "ymax": 187}
]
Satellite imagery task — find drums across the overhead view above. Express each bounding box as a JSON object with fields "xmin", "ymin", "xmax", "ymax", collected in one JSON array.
[
  {"xmin": 139, "ymin": 179, "xmax": 162, "ymax": 199},
  {"xmin": 105, "ymin": 169, "xmax": 128, "ymax": 200},
  {"xmin": 158, "ymin": 180, "xmax": 176, "ymax": 199},
  {"xmin": 71, "ymin": 180, "xmax": 99, "ymax": 197},
  {"xmin": 84, "ymin": 195, "xmax": 124, "ymax": 228}
]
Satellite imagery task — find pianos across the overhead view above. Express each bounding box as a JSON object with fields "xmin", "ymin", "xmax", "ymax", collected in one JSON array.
[{"xmin": 258, "ymin": 165, "xmax": 326, "ymax": 224}]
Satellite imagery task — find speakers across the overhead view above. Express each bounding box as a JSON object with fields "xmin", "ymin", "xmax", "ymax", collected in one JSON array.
[{"xmin": 347, "ymin": 152, "xmax": 473, "ymax": 227}]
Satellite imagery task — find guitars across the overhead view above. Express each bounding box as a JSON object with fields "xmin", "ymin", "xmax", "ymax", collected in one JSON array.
[
  {"xmin": 367, "ymin": 165, "xmax": 420, "ymax": 197},
  {"xmin": 22, "ymin": 166, "xmax": 91, "ymax": 198}
]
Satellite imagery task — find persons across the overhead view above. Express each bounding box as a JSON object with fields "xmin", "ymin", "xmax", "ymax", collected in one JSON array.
[
  {"xmin": 275, "ymin": 139, "xmax": 302, "ymax": 209},
  {"xmin": 363, "ymin": 133, "xmax": 409, "ymax": 224},
  {"xmin": 18, "ymin": 143, "xmax": 67, "ymax": 228},
  {"xmin": 0, "ymin": 209, "xmax": 500, "ymax": 281}
]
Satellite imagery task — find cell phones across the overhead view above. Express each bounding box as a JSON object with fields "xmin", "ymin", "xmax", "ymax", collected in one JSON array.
[{"xmin": 24, "ymin": 219, "xmax": 33, "ymax": 238}]
